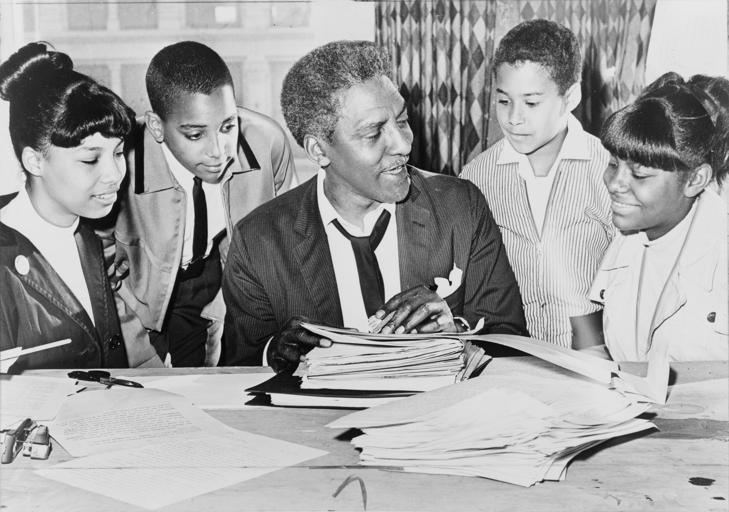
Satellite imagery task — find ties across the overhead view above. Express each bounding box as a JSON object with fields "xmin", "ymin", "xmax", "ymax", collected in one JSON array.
[
  {"xmin": 193, "ymin": 177, "xmax": 208, "ymax": 259},
  {"xmin": 331, "ymin": 213, "xmax": 392, "ymax": 321}
]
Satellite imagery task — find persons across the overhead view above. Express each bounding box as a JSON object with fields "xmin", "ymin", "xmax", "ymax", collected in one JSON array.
[
  {"xmin": 0, "ymin": 39, "xmax": 137, "ymax": 369},
  {"xmin": 94, "ymin": 40, "xmax": 300, "ymax": 367},
  {"xmin": 584, "ymin": 69, "xmax": 729, "ymax": 362},
  {"xmin": 221, "ymin": 38, "xmax": 532, "ymax": 367},
  {"xmin": 455, "ymin": 17, "xmax": 614, "ymax": 360}
]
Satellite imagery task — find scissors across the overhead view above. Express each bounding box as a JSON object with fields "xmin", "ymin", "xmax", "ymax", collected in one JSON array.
[{"xmin": 68, "ymin": 371, "xmax": 143, "ymax": 389}]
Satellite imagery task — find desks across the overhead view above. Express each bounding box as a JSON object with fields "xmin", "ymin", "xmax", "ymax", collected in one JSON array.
[{"xmin": 0, "ymin": 356, "xmax": 729, "ymax": 511}]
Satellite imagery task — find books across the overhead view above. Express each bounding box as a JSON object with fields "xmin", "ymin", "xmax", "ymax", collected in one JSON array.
[{"xmin": 244, "ymin": 355, "xmax": 493, "ymax": 410}]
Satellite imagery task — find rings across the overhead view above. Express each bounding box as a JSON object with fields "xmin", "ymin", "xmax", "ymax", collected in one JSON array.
[{"xmin": 422, "ymin": 279, "xmax": 438, "ymax": 293}]
